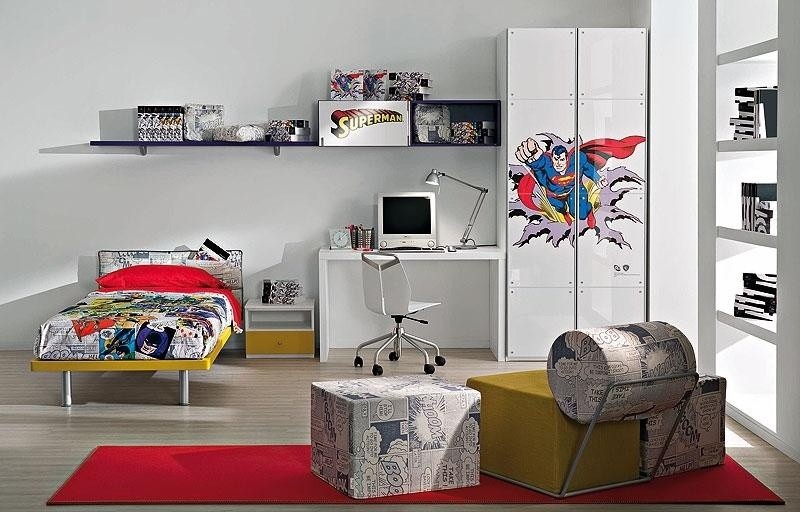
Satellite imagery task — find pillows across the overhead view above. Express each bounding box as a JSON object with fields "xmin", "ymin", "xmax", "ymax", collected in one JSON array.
[{"xmin": 95, "ymin": 265, "xmax": 225, "ymax": 290}]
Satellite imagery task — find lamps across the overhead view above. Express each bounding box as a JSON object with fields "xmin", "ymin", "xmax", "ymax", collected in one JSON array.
[{"xmin": 424, "ymin": 169, "xmax": 488, "ymax": 249}]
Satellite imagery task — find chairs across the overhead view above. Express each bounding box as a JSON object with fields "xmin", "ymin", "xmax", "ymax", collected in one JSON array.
[{"xmin": 354, "ymin": 252, "xmax": 446, "ymax": 376}]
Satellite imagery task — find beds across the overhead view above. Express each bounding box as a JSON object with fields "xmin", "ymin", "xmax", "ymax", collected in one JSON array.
[{"xmin": 29, "ymin": 249, "xmax": 243, "ymax": 407}]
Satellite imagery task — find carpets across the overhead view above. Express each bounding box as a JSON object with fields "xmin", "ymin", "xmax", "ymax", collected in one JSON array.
[{"xmin": 46, "ymin": 445, "xmax": 786, "ymax": 506}]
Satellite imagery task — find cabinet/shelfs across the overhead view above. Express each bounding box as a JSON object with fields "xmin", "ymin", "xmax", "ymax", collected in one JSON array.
[
  {"xmin": 716, "ymin": 37, "xmax": 778, "ymax": 345},
  {"xmin": 495, "ymin": 25, "xmax": 649, "ymax": 361}
]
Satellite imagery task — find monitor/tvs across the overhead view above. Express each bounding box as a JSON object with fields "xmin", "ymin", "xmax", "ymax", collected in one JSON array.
[{"xmin": 378, "ymin": 192, "xmax": 437, "ymax": 250}]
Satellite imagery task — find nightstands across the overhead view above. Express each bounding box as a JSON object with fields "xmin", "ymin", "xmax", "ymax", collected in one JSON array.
[{"xmin": 244, "ymin": 296, "xmax": 315, "ymax": 358}]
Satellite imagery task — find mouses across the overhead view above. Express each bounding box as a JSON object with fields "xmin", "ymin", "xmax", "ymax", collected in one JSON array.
[{"xmin": 448, "ymin": 245, "xmax": 456, "ymax": 252}]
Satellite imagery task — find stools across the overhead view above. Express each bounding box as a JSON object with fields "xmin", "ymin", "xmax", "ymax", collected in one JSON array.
[
  {"xmin": 466, "ymin": 370, "xmax": 640, "ymax": 494},
  {"xmin": 641, "ymin": 374, "xmax": 726, "ymax": 478},
  {"xmin": 310, "ymin": 378, "xmax": 480, "ymax": 499}
]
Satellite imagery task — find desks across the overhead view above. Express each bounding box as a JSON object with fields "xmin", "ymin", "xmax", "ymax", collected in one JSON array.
[{"xmin": 319, "ymin": 246, "xmax": 506, "ymax": 363}]
